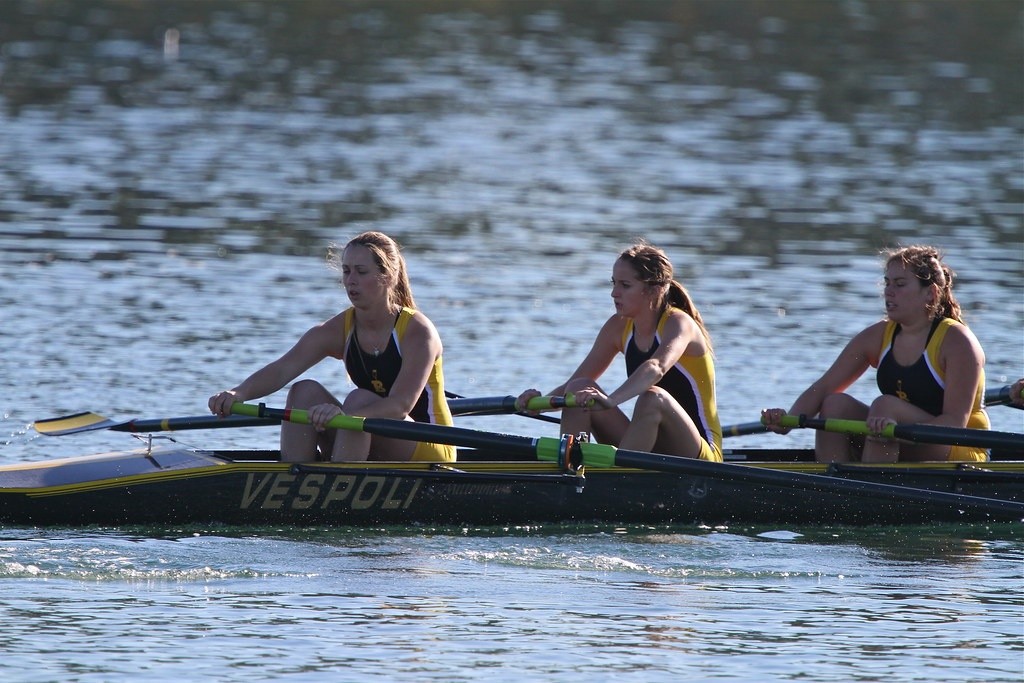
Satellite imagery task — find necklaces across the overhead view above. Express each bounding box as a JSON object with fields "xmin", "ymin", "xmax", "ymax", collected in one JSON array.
[
  {"xmin": 634, "ymin": 324, "xmax": 653, "ymax": 352},
  {"xmin": 364, "ymin": 309, "xmax": 393, "ymax": 356}
]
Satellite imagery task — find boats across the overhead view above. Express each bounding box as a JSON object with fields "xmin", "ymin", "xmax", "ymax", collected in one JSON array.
[{"xmin": 0, "ymin": 426, "xmax": 1024, "ymax": 534}]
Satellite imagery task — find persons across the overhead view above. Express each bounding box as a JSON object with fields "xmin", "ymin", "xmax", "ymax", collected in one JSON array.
[
  {"xmin": 760, "ymin": 245, "xmax": 1024, "ymax": 464},
  {"xmin": 208, "ymin": 231, "xmax": 460, "ymax": 463},
  {"xmin": 517, "ymin": 243, "xmax": 723, "ymax": 463}
]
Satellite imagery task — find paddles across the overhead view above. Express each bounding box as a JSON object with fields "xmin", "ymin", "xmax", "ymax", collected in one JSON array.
[
  {"xmin": 760, "ymin": 413, "xmax": 1024, "ymax": 451},
  {"xmin": 220, "ymin": 398, "xmax": 1024, "ymax": 515},
  {"xmin": 720, "ymin": 384, "xmax": 1024, "ymax": 439},
  {"xmin": 32, "ymin": 391, "xmax": 595, "ymax": 437}
]
